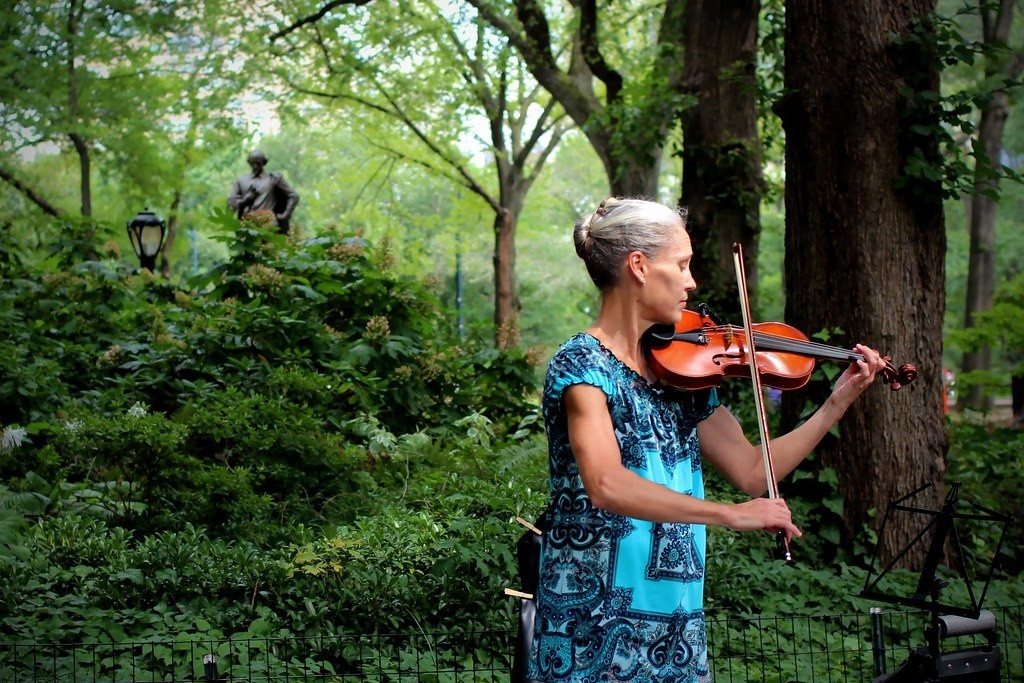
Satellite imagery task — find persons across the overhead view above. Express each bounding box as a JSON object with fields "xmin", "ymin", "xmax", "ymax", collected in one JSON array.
[
  {"xmin": 225, "ymin": 150, "xmax": 300, "ymax": 235},
  {"xmin": 523, "ymin": 197, "xmax": 885, "ymax": 683}
]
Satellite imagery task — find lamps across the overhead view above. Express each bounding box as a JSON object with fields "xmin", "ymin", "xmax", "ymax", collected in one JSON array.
[{"xmin": 127, "ymin": 207, "xmax": 167, "ymax": 260}]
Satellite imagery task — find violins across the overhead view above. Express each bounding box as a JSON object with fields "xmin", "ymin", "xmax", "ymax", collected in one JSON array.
[{"xmin": 645, "ymin": 308, "xmax": 917, "ymax": 390}]
